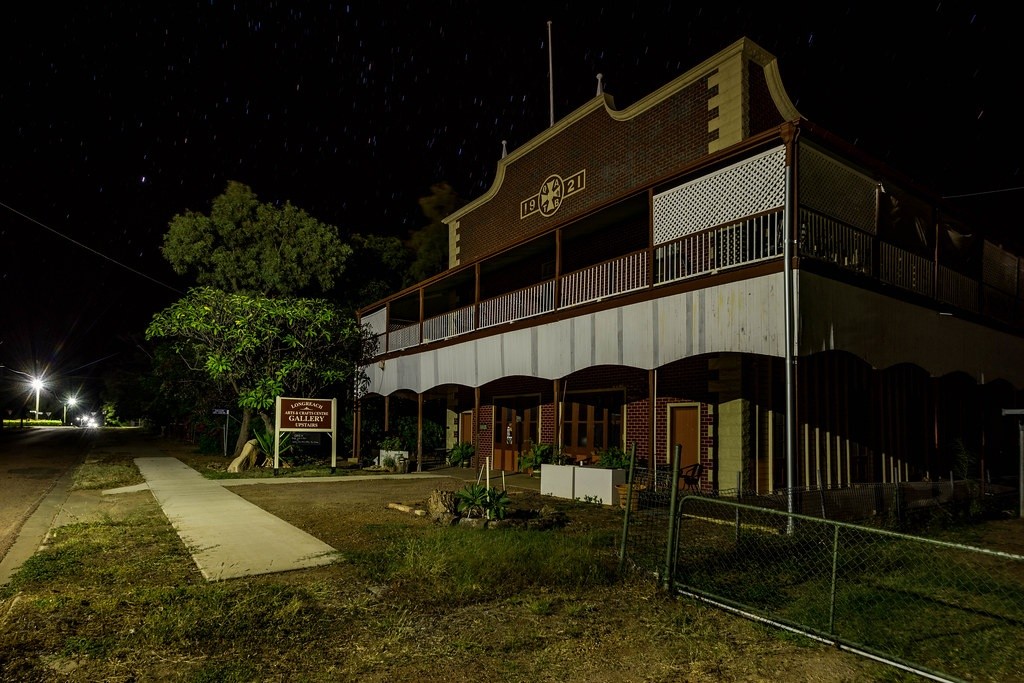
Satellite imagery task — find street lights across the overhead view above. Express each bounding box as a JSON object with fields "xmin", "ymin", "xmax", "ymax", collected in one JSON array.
[
  {"xmin": 63, "ymin": 396, "xmax": 77, "ymax": 425},
  {"xmin": 31, "ymin": 379, "xmax": 44, "ymax": 420}
]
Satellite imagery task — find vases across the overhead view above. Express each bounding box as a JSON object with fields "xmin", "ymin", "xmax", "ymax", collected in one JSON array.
[{"xmin": 615, "ymin": 483, "xmax": 646, "ymax": 510}]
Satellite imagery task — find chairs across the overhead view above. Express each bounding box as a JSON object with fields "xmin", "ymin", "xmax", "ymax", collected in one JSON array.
[{"xmin": 680, "ymin": 463, "xmax": 705, "ymax": 498}]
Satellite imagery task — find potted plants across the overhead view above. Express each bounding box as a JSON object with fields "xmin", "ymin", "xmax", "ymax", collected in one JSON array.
[
  {"xmin": 450, "ymin": 441, "xmax": 474, "ymax": 467},
  {"xmin": 381, "ymin": 454, "xmax": 396, "ymax": 473},
  {"xmin": 517, "ymin": 438, "xmax": 552, "ymax": 479}
]
